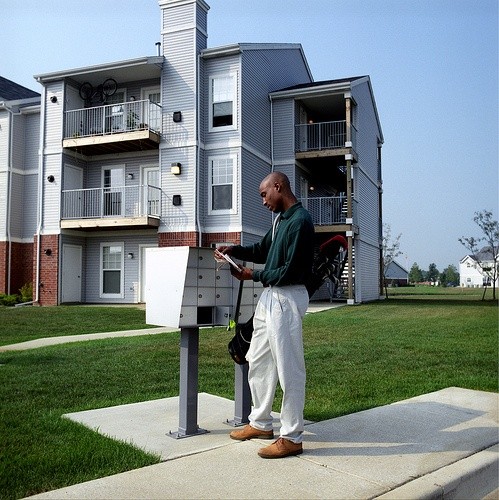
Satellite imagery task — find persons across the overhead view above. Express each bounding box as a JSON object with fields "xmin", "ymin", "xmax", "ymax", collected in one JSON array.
[{"xmin": 215, "ymin": 172, "xmax": 315, "ymax": 459}]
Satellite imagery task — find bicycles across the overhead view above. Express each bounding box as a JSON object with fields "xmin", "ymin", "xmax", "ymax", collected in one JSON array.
[{"xmin": 78, "ymin": 79, "xmax": 117, "ymax": 108}]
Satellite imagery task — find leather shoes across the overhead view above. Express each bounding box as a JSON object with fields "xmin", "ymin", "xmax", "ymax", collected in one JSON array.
[
  {"xmin": 229, "ymin": 423, "xmax": 274, "ymax": 440},
  {"xmin": 258, "ymin": 437, "xmax": 303, "ymax": 459}
]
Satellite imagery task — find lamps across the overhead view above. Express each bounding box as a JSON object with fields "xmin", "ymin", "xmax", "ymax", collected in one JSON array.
[
  {"xmin": 129, "ymin": 96, "xmax": 135, "ymax": 101},
  {"xmin": 128, "ymin": 173, "xmax": 134, "ymax": 179},
  {"xmin": 170, "ymin": 162, "xmax": 181, "ymax": 175},
  {"xmin": 127, "ymin": 252, "xmax": 134, "ymax": 259}
]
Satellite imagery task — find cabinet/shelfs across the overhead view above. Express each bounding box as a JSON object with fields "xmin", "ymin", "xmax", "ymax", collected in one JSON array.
[{"xmin": 145, "ymin": 246, "xmax": 266, "ymax": 440}]
